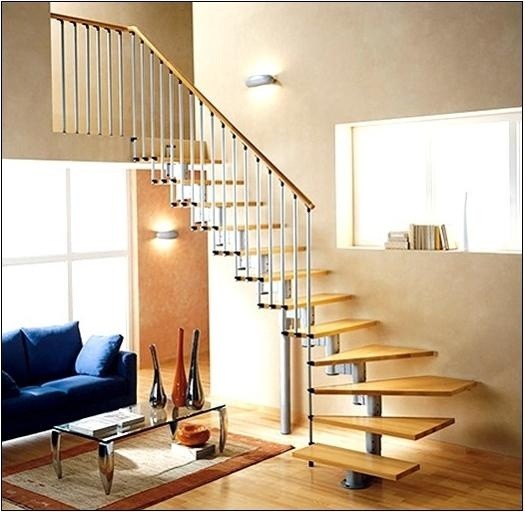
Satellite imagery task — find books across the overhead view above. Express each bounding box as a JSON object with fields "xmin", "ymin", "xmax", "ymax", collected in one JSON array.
[
  {"xmin": 69, "ymin": 408, "xmax": 145, "ymax": 439},
  {"xmin": 385, "ymin": 223, "xmax": 458, "ymax": 250}
]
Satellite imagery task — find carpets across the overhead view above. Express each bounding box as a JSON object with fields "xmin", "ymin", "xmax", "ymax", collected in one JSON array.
[{"xmin": 1, "ymin": 422, "xmax": 295, "ymax": 510}]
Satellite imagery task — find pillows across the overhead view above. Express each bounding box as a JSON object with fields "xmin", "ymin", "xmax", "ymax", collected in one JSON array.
[{"xmin": 2, "ymin": 321, "xmax": 123, "ymax": 396}]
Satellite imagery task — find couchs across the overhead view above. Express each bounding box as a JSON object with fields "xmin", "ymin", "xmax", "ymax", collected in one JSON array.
[{"xmin": 2, "ymin": 320, "xmax": 137, "ymax": 439}]
